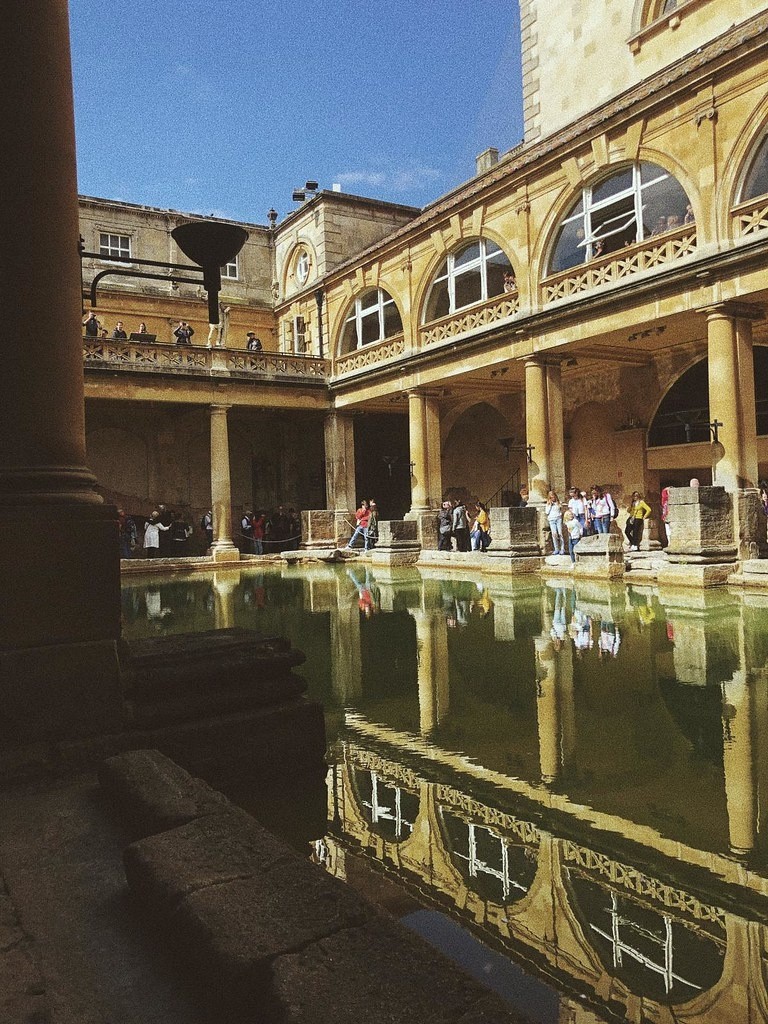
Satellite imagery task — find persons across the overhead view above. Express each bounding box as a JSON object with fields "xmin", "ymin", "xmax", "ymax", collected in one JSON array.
[
  {"xmin": 503, "ymin": 270, "xmax": 520, "ymax": 294},
  {"xmin": 174, "ymin": 320, "xmax": 195, "ymax": 364},
  {"xmin": 246, "ymin": 330, "xmax": 263, "ymax": 371},
  {"xmin": 208, "ymin": 299, "xmax": 231, "ymax": 346},
  {"xmin": 624, "ymin": 491, "xmax": 652, "ymax": 552},
  {"xmin": 587, "ymin": 233, "xmax": 606, "ymax": 260},
  {"xmin": 201, "ymin": 507, "xmax": 212, "ymax": 547},
  {"xmin": 144, "ymin": 505, "xmax": 192, "ymax": 558},
  {"xmin": 625, "ymin": 202, "xmax": 695, "ymax": 246},
  {"xmin": 549, "ymin": 587, "xmax": 620, "ymax": 663},
  {"xmin": 139, "ymin": 322, "xmax": 149, "ymax": 335},
  {"xmin": 544, "ymin": 486, "xmax": 619, "ymax": 564},
  {"xmin": 661, "ymin": 487, "xmax": 675, "ymax": 549},
  {"xmin": 117, "ymin": 508, "xmax": 138, "ymax": 559},
  {"xmin": 343, "ymin": 564, "xmax": 380, "ymax": 620},
  {"xmin": 440, "ymin": 580, "xmax": 491, "ymax": 633},
  {"xmin": 112, "ymin": 320, "xmax": 129, "ymax": 362},
  {"xmin": 132, "ymin": 582, "xmax": 214, "ymax": 634},
  {"xmin": 638, "ymin": 597, "xmax": 656, "ymax": 630},
  {"xmin": 242, "ymin": 506, "xmax": 302, "ymax": 556},
  {"xmin": 82, "ymin": 309, "xmax": 107, "ymax": 360},
  {"xmin": 439, "ymin": 498, "xmax": 493, "ymax": 553},
  {"xmin": 244, "ymin": 569, "xmax": 292, "ymax": 613},
  {"xmin": 345, "ymin": 499, "xmax": 378, "ymax": 552}
]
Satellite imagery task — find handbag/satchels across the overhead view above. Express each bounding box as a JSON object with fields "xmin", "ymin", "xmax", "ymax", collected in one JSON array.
[
  {"xmin": 605, "ymin": 493, "xmax": 619, "ymax": 519},
  {"xmin": 626, "ymin": 516, "xmax": 635, "ymax": 525},
  {"xmin": 485, "ymin": 534, "xmax": 491, "ymax": 547}
]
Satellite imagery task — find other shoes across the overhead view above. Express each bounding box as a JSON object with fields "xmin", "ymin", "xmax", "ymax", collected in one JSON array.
[
  {"xmin": 558, "ymin": 550, "xmax": 565, "ymax": 555},
  {"xmin": 628, "ymin": 544, "xmax": 639, "ymax": 552},
  {"xmin": 552, "ymin": 550, "xmax": 558, "ymax": 555}
]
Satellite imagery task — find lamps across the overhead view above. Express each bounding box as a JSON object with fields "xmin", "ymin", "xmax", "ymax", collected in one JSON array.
[
  {"xmin": 500, "ymin": 367, "xmax": 510, "ymax": 376},
  {"xmin": 380, "ymin": 455, "xmax": 415, "ymax": 478},
  {"xmin": 655, "ymin": 324, "xmax": 667, "ymax": 336},
  {"xmin": 498, "ymin": 432, "xmax": 536, "ymax": 464},
  {"xmin": 292, "ymin": 189, "xmax": 319, "ymax": 202},
  {"xmin": 641, "ymin": 329, "xmax": 653, "ymax": 339},
  {"xmin": 673, "ymin": 409, "xmax": 725, "ymax": 444},
  {"xmin": 490, "ymin": 370, "xmax": 500, "ymax": 379},
  {"xmin": 305, "ymin": 179, "xmax": 319, "ymax": 193},
  {"xmin": 566, "ymin": 358, "xmax": 582, "ymax": 367},
  {"xmin": 627, "ymin": 332, "xmax": 643, "ymax": 342},
  {"xmin": 79, "ymin": 220, "xmax": 249, "ymax": 325}
]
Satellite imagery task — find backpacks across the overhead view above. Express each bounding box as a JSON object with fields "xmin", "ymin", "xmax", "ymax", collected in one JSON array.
[{"xmin": 200, "ymin": 515, "xmax": 212, "ymax": 530}]
